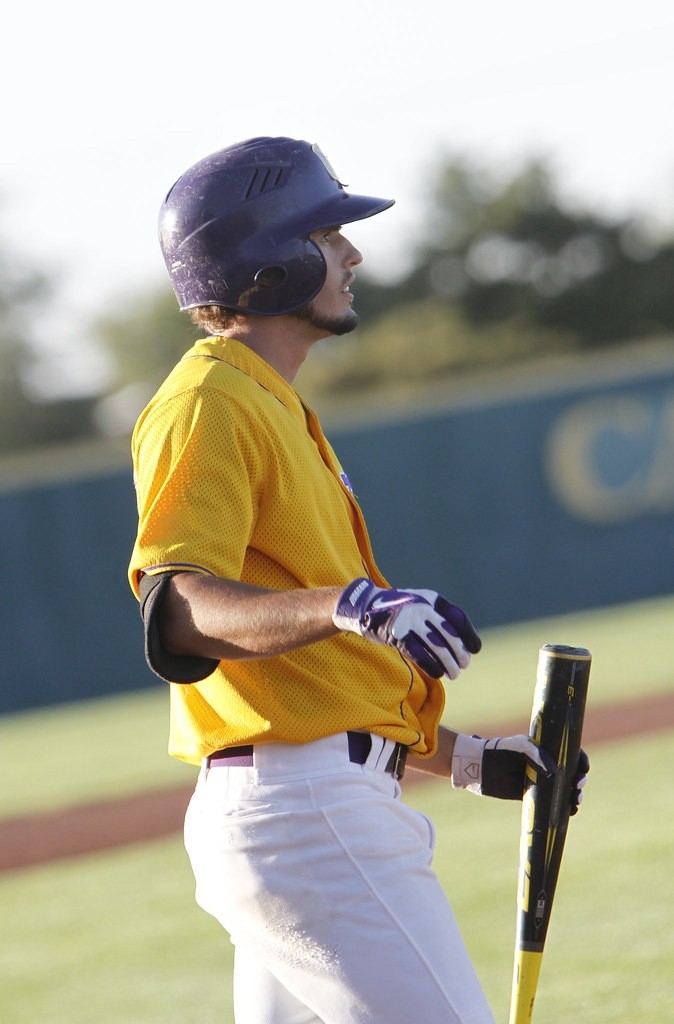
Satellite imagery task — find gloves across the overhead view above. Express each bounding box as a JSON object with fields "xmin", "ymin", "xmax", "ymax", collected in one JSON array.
[
  {"xmin": 448, "ymin": 730, "xmax": 590, "ymax": 819},
  {"xmin": 331, "ymin": 573, "xmax": 480, "ymax": 682}
]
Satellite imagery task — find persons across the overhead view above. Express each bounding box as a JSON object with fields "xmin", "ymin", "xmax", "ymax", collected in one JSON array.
[{"xmin": 126, "ymin": 135, "xmax": 590, "ymax": 1024}]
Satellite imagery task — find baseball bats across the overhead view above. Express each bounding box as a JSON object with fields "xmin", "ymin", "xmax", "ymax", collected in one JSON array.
[{"xmin": 510, "ymin": 641, "xmax": 593, "ymax": 1024}]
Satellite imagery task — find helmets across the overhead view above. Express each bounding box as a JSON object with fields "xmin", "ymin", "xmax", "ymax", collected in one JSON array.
[{"xmin": 158, "ymin": 133, "xmax": 399, "ymax": 325}]
